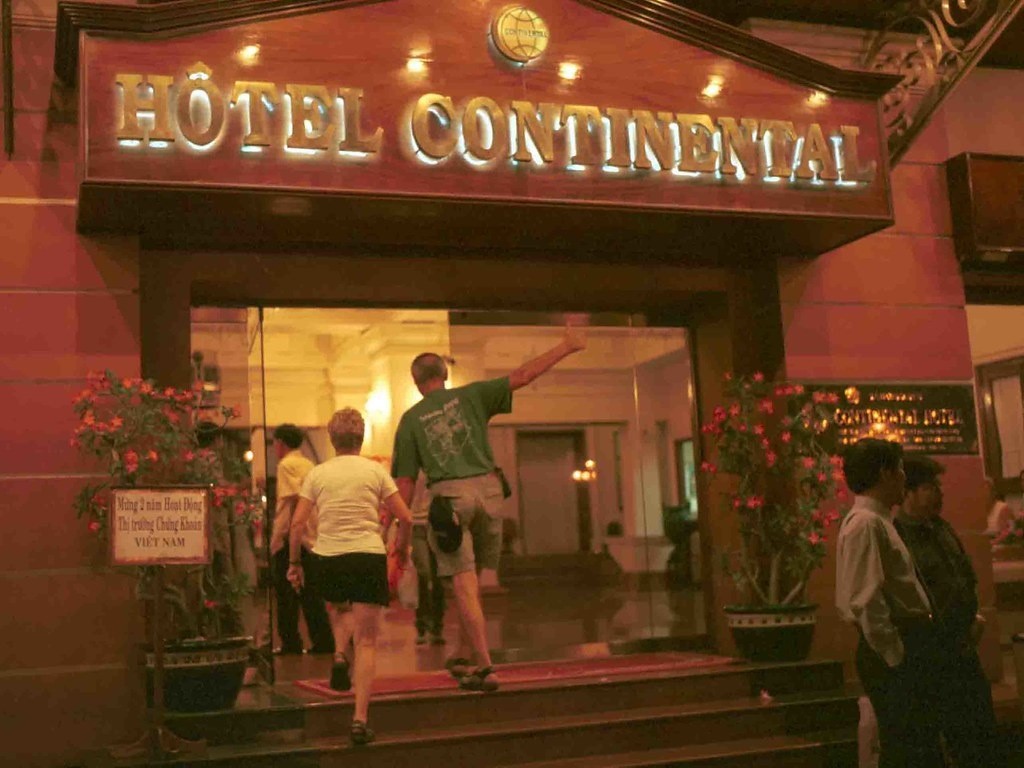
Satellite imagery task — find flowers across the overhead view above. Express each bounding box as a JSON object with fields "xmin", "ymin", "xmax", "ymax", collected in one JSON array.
[
  {"xmin": 699, "ymin": 364, "xmax": 833, "ymax": 604},
  {"xmin": 73, "ymin": 368, "xmax": 266, "ymax": 641}
]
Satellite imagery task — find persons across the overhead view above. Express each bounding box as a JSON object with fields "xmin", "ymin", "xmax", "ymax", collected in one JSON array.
[
  {"xmin": 194, "ymin": 405, "xmax": 447, "ymax": 744},
  {"xmin": 834, "ymin": 437, "xmax": 1014, "ymax": 768},
  {"xmin": 393, "ymin": 320, "xmax": 588, "ymax": 691}
]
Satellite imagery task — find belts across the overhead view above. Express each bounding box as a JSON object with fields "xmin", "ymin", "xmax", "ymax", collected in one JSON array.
[{"xmin": 889, "ymin": 611, "xmax": 934, "ymax": 626}]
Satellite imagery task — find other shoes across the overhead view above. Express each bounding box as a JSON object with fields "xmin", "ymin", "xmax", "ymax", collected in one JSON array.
[
  {"xmin": 271, "ymin": 645, "xmax": 302, "ymax": 655},
  {"xmin": 307, "ymin": 644, "xmax": 335, "ymax": 654},
  {"xmin": 415, "ymin": 633, "xmax": 427, "ymax": 644},
  {"xmin": 430, "ymin": 632, "xmax": 446, "ymax": 645}
]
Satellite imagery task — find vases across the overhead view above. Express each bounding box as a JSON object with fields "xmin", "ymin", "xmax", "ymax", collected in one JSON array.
[
  {"xmin": 143, "ymin": 645, "xmax": 245, "ymax": 713},
  {"xmin": 728, "ymin": 606, "xmax": 818, "ymax": 661}
]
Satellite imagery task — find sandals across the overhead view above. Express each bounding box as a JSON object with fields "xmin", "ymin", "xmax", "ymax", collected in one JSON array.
[
  {"xmin": 331, "ymin": 653, "xmax": 351, "ymax": 691},
  {"xmin": 350, "ymin": 722, "xmax": 376, "ymax": 743},
  {"xmin": 459, "ymin": 666, "xmax": 499, "ymax": 693},
  {"xmin": 449, "ymin": 659, "xmax": 481, "ymax": 678}
]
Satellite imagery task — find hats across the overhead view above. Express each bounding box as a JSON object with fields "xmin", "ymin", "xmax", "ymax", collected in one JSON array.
[{"xmin": 429, "ymin": 495, "xmax": 464, "ymax": 553}]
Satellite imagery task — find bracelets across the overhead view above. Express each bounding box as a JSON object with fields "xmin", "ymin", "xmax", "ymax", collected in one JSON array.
[{"xmin": 289, "ymin": 561, "xmax": 301, "ymax": 566}]
